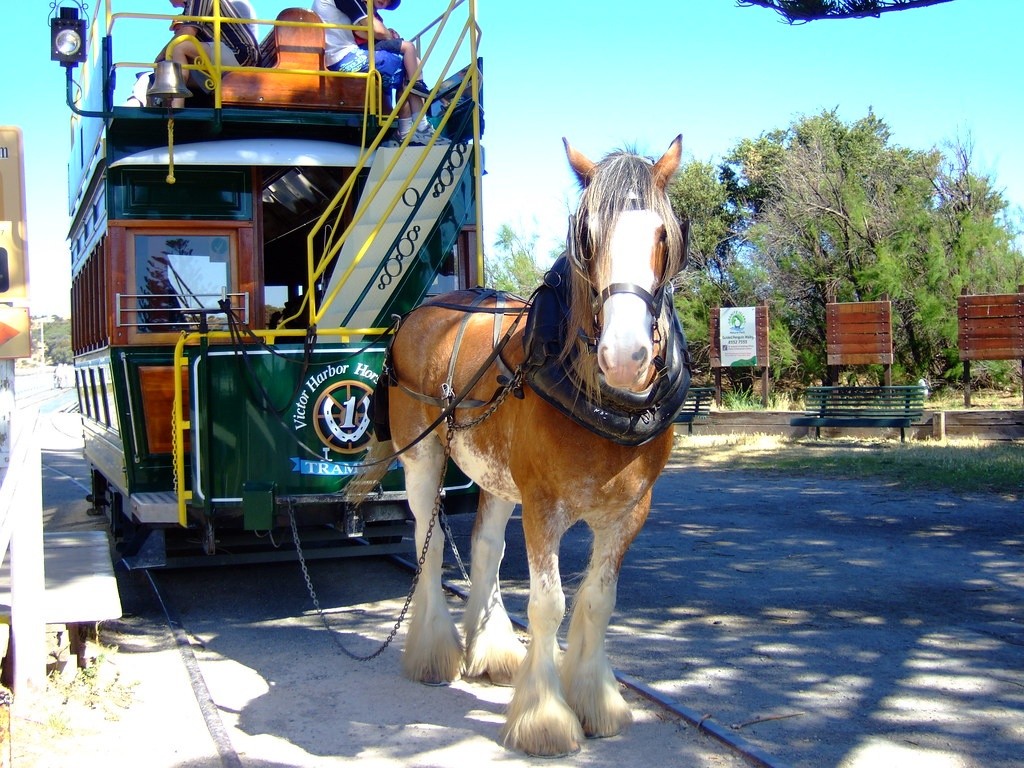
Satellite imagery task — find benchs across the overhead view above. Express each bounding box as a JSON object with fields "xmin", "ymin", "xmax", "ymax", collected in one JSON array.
[
  {"xmin": 673, "ymin": 387, "xmax": 716, "ymax": 437},
  {"xmin": 206, "ymin": 8, "xmax": 384, "ymax": 113},
  {"xmin": 790, "ymin": 386, "xmax": 926, "ymax": 443}
]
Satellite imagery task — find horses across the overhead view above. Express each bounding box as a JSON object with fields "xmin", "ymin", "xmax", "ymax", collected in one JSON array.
[{"xmin": 325, "ymin": 133, "xmax": 693, "ymax": 760}]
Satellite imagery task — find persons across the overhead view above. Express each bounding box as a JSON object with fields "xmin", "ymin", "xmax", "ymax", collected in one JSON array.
[{"xmin": 120, "ymin": 0, "xmax": 453, "ymax": 148}]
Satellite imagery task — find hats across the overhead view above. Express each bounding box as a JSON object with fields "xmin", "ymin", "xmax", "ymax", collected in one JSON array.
[{"xmin": 169, "ymin": 20, "xmax": 183, "ymax": 31}]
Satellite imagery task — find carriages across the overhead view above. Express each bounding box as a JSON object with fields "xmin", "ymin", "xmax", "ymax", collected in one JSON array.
[{"xmin": 47, "ymin": 0, "xmax": 695, "ymax": 761}]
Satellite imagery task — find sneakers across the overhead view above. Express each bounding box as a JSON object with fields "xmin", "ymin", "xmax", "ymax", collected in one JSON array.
[
  {"xmin": 403, "ymin": 79, "xmax": 436, "ymax": 99},
  {"xmin": 417, "ymin": 120, "xmax": 452, "ymax": 145},
  {"xmin": 392, "ymin": 130, "xmax": 427, "ymax": 146}
]
